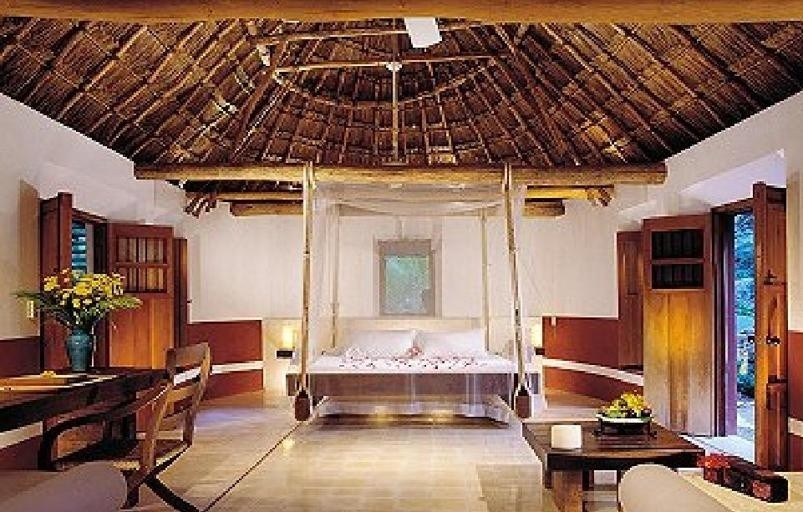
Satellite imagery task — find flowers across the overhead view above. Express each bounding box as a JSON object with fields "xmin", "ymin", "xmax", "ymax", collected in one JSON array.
[
  {"xmin": 10, "ymin": 268, "xmax": 145, "ymax": 338},
  {"xmin": 599, "ymin": 392, "xmax": 655, "ymax": 419}
]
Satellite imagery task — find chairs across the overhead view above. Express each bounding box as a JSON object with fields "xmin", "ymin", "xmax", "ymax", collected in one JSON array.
[{"xmin": 37, "ymin": 340, "xmax": 213, "ymax": 512}]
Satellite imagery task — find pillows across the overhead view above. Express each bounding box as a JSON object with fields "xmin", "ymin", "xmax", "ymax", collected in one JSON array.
[
  {"xmin": 341, "ymin": 329, "xmax": 417, "ymax": 358},
  {"xmin": 418, "ymin": 324, "xmax": 487, "ymax": 357}
]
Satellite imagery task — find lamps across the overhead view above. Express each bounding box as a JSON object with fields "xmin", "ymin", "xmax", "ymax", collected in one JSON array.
[
  {"xmin": 531, "ymin": 324, "xmax": 544, "ymax": 356},
  {"xmin": 275, "ymin": 324, "xmax": 296, "ymax": 359}
]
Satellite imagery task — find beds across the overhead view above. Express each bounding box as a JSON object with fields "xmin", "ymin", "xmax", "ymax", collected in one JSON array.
[{"xmin": 287, "ymin": 350, "xmax": 542, "ymax": 400}]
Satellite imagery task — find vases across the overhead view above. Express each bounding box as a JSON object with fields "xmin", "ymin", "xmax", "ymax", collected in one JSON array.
[
  {"xmin": 593, "ymin": 415, "xmax": 661, "ymax": 438},
  {"xmin": 63, "ymin": 327, "xmax": 97, "ymax": 371}
]
